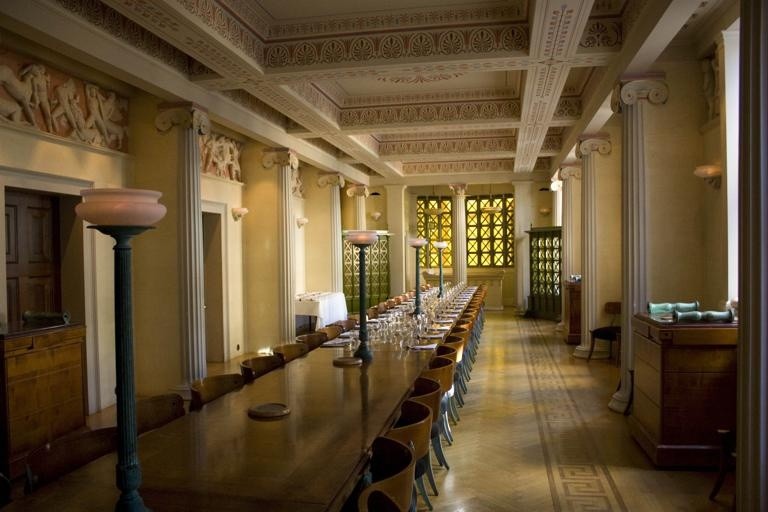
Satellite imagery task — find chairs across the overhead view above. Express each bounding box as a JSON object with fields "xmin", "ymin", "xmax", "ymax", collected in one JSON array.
[{"xmin": 586, "ymin": 298, "xmax": 622, "ymax": 365}]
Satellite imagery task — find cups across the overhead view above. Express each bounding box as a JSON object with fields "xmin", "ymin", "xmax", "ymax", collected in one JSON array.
[{"xmin": 368, "ymin": 280, "xmax": 465, "ymax": 349}]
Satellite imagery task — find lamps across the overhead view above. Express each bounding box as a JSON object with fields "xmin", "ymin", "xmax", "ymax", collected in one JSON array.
[
  {"xmin": 408, "ymin": 236, "xmax": 428, "ymax": 315},
  {"xmin": 481, "ymin": 184, "xmax": 501, "ymax": 214},
  {"xmin": 695, "ymin": 165, "xmax": 724, "ymax": 183},
  {"xmin": 422, "ymin": 185, "xmax": 444, "ymax": 216},
  {"xmin": 72, "ymin": 186, "xmax": 171, "ymax": 507},
  {"xmin": 296, "ymin": 216, "xmax": 309, "ymax": 227},
  {"xmin": 341, "ymin": 228, "xmax": 377, "ymax": 360},
  {"xmin": 538, "ymin": 207, "xmax": 551, "ymax": 215},
  {"xmin": 370, "ymin": 210, "xmax": 382, "ymax": 220},
  {"xmin": 433, "ymin": 241, "xmax": 449, "ymax": 300},
  {"xmin": 229, "ymin": 204, "xmax": 250, "ymax": 223}
]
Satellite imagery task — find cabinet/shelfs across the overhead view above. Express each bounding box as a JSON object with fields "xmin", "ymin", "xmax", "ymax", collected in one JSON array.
[
  {"xmin": 562, "ymin": 278, "xmax": 580, "ymax": 345},
  {"xmin": 625, "ymin": 312, "xmax": 736, "ymax": 467}
]
